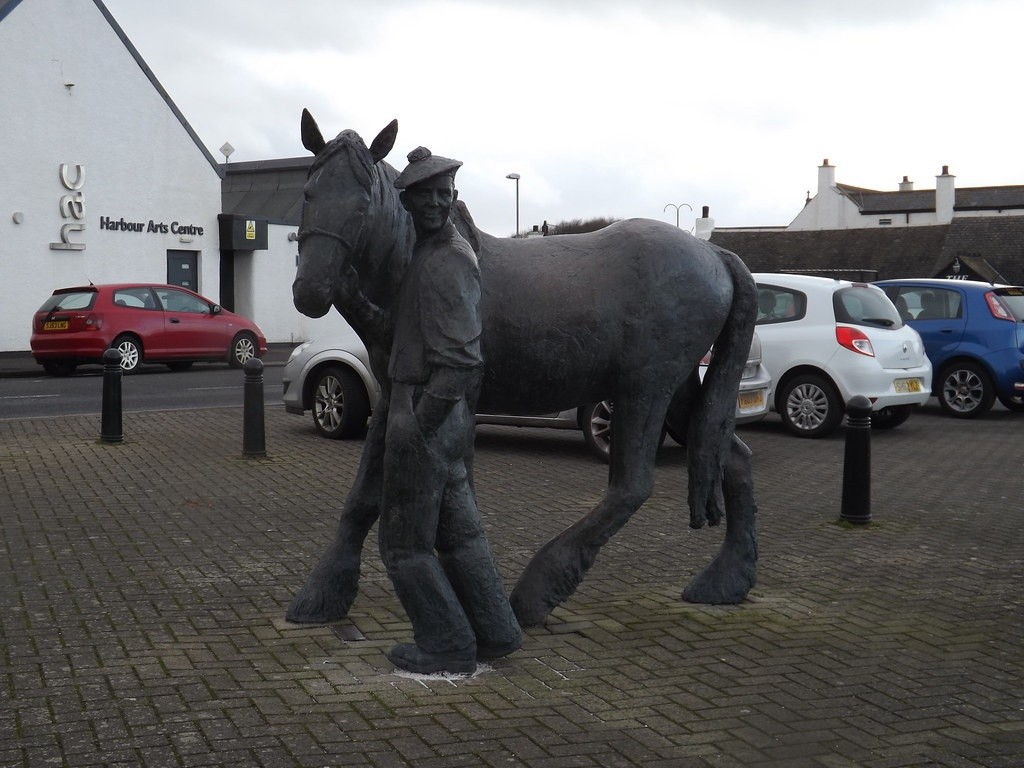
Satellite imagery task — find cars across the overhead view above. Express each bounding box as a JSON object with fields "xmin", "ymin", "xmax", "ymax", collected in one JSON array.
[
  {"xmin": 751, "ymin": 272, "xmax": 932, "ymax": 437},
  {"xmin": 869, "ymin": 279, "xmax": 1024, "ymax": 419},
  {"xmin": 282, "ymin": 329, "xmax": 772, "ymax": 458},
  {"xmin": 30, "ymin": 282, "xmax": 267, "ymax": 375}
]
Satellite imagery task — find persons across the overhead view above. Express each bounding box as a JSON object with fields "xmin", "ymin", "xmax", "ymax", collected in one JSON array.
[
  {"xmin": 915, "ymin": 291, "xmax": 945, "ymax": 320},
  {"xmin": 379, "ymin": 146, "xmax": 526, "ymax": 675}
]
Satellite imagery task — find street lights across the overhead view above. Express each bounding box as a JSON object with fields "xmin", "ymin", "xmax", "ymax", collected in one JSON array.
[{"xmin": 507, "ymin": 173, "xmax": 520, "ymax": 238}]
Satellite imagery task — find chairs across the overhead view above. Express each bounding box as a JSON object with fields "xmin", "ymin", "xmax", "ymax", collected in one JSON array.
[
  {"xmin": 757, "ymin": 290, "xmax": 779, "ymax": 322},
  {"xmin": 915, "ymin": 294, "xmax": 945, "ymax": 318},
  {"xmin": 145, "ymin": 295, "xmax": 155, "ymax": 309},
  {"xmin": 893, "ymin": 295, "xmax": 915, "ymax": 321}
]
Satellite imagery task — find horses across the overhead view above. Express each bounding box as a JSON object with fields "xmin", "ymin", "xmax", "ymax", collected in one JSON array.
[{"xmin": 286, "ymin": 109, "xmax": 758, "ymax": 628}]
